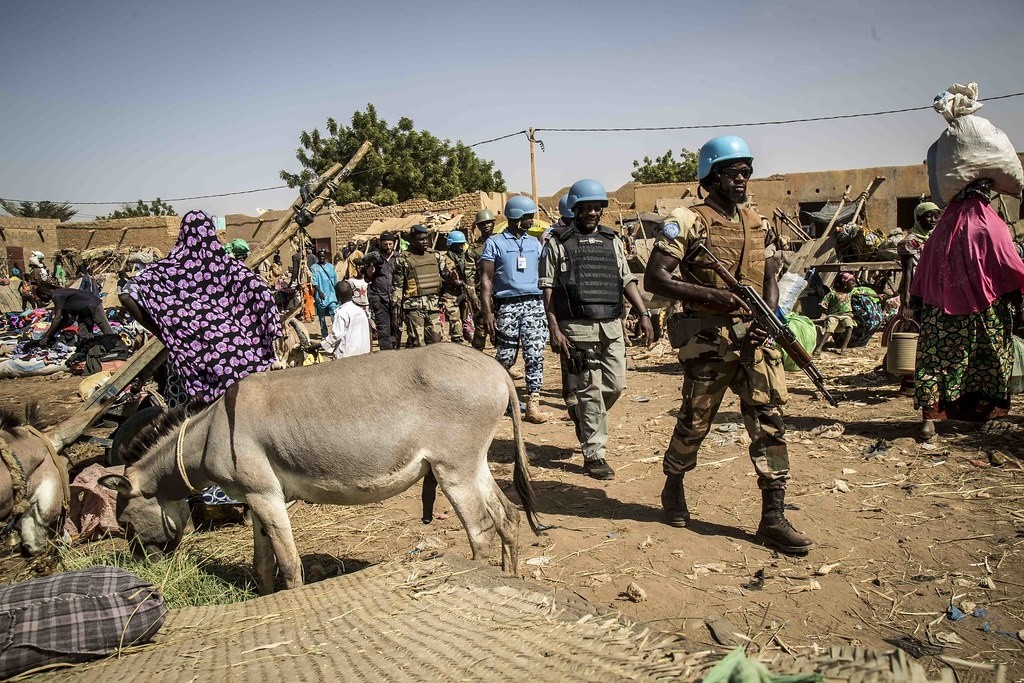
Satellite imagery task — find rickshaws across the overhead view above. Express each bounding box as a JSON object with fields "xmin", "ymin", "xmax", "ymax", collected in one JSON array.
[
  {"xmin": 75, "ymin": 140, "xmax": 373, "ymax": 467},
  {"xmin": 787, "ymin": 181, "xmax": 874, "ymax": 319}
]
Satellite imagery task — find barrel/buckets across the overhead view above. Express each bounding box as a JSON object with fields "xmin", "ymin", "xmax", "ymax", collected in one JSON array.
[{"xmin": 887, "ymin": 318, "xmax": 920, "ymax": 374}]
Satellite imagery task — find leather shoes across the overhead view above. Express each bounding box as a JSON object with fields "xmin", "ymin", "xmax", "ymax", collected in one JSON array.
[
  {"xmin": 584, "ymin": 458, "xmax": 615, "ymax": 479},
  {"xmin": 568, "ymin": 405, "xmax": 581, "ymax": 440}
]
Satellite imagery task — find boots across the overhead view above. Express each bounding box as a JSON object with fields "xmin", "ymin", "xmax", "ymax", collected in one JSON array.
[
  {"xmin": 523, "ymin": 393, "xmax": 547, "ymax": 423},
  {"xmin": 754, "ymin": 488, "xmax": 815, "ymax": 553},
  {"xmin": 662, "ymin": 475, "xmax": 689, "ymax": 526}
]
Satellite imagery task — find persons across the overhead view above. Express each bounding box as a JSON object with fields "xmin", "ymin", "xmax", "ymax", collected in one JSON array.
[
  {"xmin": 12, "ymin": 262, "xmax": 23, "ymax": 280},
  {"xmin": 813, "ymin": 272, "xmax": 886, "ymax": 359},
  {"xmin": 897, "ymin": 201, "xmax": 940, "ymax": 396},
  {"xmin": 643, "ymin": 134, "xmax": 815, "ymax": 552},
  {"xmin": 259, "ymin": 255, "xmax": 281, "ymax": 279},
  {"xmin": 909, "ymin": 177, "xmax": 1024, "ymax": 439},
  {"xmin": 54, "ymin": 255, "xmax": 65, "ymax": 285},
  {"xmin": 35, "ymin": 282, "xmax": 114, "ymax": 346},
  {"xmin": 537, "ymin": 179, "xmax": 654, "ymax": 479},
  {"xmin": 292, "ymin": 195, "xmax": 549, "ymax": 423},
  {"xmin": 118, "ymin": 209, "xmax": 283, "ymax": 525},
  {"xmin": 18, "ymin": 274, "xmax": 36, "ymax": 310},
  {"xmin": 79, "ymin": 259, "xmax": 93, "ymax": 276},
  {"xmin": 29, "ymin": 264, "xmax": 48, "ymax": 281}
]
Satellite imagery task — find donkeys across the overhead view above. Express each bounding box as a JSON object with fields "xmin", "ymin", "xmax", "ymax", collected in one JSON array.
[
  {"xmin": 0, "ymin": 399, "xmax": 71, "ymax": 577},
  {"xmin": 279, "ymin": 303, "xmax": 323, "ymax": 368},
  {"xmin": 96, "ymin": 343, "xmax": 543, "ymax": 597}
]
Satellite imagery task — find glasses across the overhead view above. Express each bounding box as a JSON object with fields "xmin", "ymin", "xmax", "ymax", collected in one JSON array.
[{"xmin": 715, "ymin": 167, "xmax": 752, "ymax": 178}]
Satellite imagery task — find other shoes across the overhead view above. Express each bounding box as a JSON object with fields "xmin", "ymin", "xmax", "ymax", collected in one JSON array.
[
  {"xmin": 813, "ymin": 351, "xmax": 820, "ymax": 359},
  {"xmin": 562, "ymin": 391, "xmax": 578, "ymax": 406},
  {"xmin": 920, "ymin": 420, "xmax": 935, "ymax": 438}
]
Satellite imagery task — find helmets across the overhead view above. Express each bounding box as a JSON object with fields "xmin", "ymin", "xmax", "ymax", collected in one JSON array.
[
  {"xmin": 566, "ymin": 179, "xmax": 608, "ymax": 209},
  {"xmin": 698, "ymin": 136, "xmax": 753, "ymax": 180},
  {"xmin": 475, "ymin": 210, "xmax": 496, "ymax": 224},
  {"xmin": 505, "ymin": 196, "xmax": 538, "ymax": 219},
  {"xmin": 559, "ymin": 195, "xmax": 574, "ymax": 218},
  {"xmin": 446, "ymin": 231, "xmax": 466, "ymax": 246}
]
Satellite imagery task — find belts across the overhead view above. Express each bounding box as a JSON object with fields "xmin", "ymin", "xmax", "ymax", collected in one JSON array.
[{"xmin": 494, "ymin": 295, "xmax": 542, "ymax": 303}]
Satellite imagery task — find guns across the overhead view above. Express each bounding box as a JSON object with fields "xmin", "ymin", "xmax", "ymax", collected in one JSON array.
[
  {"xmin": 683, "ymin": 241, "xmax": 846, "ymax": 410},
  {"xmin": 452, "ymin": 265, "xmax": 478, "ymax": 314}
]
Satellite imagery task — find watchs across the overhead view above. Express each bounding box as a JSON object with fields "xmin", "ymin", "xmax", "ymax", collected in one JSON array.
[{"xmin": 640, "ymin": 309, "xmax": 651, "ymax": 318}]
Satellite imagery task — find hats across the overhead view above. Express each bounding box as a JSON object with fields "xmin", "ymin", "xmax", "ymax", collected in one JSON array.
[
  {"xmin": 380, "ymin": 232, "xmax": 395, "ymax": 242},
  {"xmin": 410, "ymin": 225, "xmax": 428, "ymax": 234}
]
